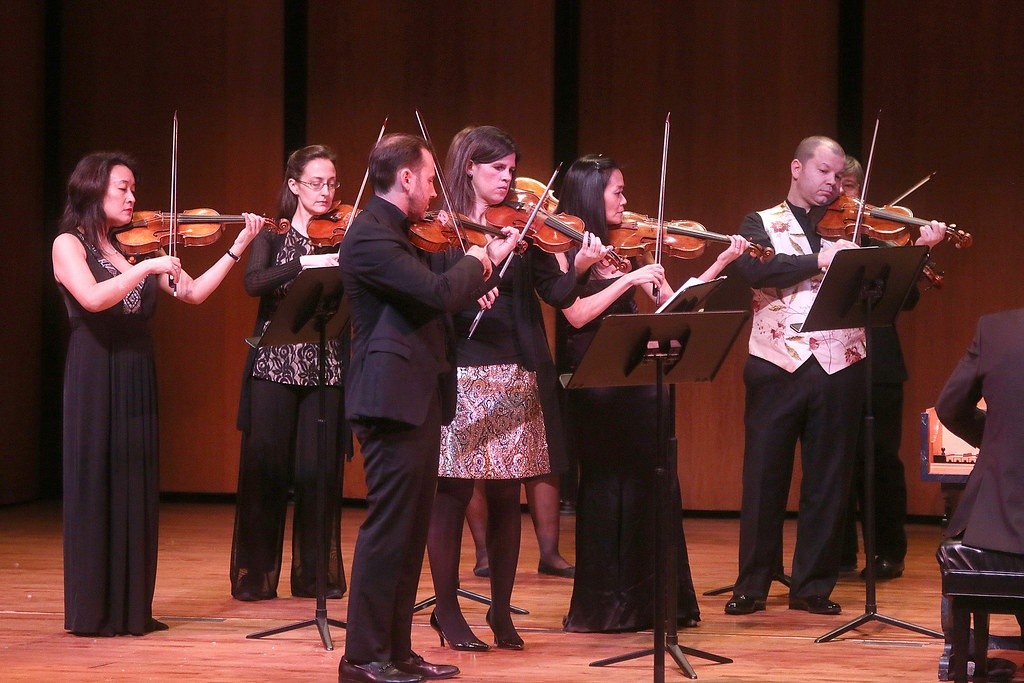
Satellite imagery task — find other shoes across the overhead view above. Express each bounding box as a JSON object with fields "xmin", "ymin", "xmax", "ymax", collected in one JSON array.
[
  {"xmin": 538, "ymin": 561, "xmax": 575, "ymax": 578},
  {"xmin": 326, "ymin": 589, "xmax": 343, "ymax": 599},
  {"xmin": 148, "ymin": 618, "xmax": 169, "ymax": 632},
  {"xmin": 677, "ymin": 616, "xmax": 697, "ymax": 627},
  {"xmin": 239, "ymin": 592, "xmax": 260, "ymax": 602},
  {"xmin": 474, "ymin": 566, "xmax": 491, "ymax": 577}
]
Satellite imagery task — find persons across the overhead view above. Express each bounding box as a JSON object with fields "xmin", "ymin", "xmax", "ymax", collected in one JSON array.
[
  {"xmin": 428, "ymin": 125, "xmax": 613, "ymax": 651},
  {"xmin": 839, "ymin": 156, "xmax": 920, "ymax": 578},
  {"xmin": 936, "ymin": 308, "xmax": 1024, "ymax": 556},
  {"xmin": 559, "ymin": 154, "xmax": 751, "ymax": 633},
  {"xmin": 339, "ymin": 133, "xmax": 520, "ymax": 683},
  {"xmin": 465, "ymin": 477, "xmax": 575, "ymax": 579},
  {"xmin": 230, "ymin": 145, "xmax": 354, "ymax": 602},
  {"xmin": 52, "ymin": 152, "xmax": 265, "ymax": 636},
  {"xmin": 723, "ymin": 136, "xmax": 946, "ymax": 614}
]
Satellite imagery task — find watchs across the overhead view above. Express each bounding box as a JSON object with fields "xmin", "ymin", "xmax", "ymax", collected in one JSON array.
[{"xmin": 227, "ymin": 250, "xmax": 241, "ymax": 262}]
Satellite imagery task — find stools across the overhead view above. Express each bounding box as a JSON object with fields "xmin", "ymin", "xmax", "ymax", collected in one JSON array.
[{"xmin": 938, "ymin": 543, "xmax": 1024, "ymax": 683}]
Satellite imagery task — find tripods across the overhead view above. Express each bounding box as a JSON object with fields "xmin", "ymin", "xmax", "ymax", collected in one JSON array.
[
  {"xmin": 790, "ymin": 245, "xmax": 947, "ymax": 643},
  {"xmin": 246, "ymin": 266, "xmax": 353, "ymax": 652},
  {"xmin": 413, "ymin": 563, "xmax": 534, "ymax": 617},
  {"xmin": 560, "ymin": 311, "xmax": 752, "ymax": 679}
]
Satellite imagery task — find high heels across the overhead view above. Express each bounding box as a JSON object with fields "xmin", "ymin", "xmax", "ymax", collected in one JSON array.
[
  {"xmin": 486, "ymin": 605, "xmax": 525, "ymax": 649},
  {"xmin": 430, "ymin": 607, "xmax": 491, "ymax": 651}
]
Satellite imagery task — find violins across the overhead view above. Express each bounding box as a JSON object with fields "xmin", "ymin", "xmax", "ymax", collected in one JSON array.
[
  {"xmin": 114, "ymin": 207, "xmax": 292, "ymax": 256},
  {"xmin": 307, "ymin": 203, "xmax": 363, "ymax": 247},
  {"xmin": 406, "ymin": 209, "xmax": 529, "ymax": 256},
  {"xmin": 605, "ymin": 210, "xmax": 775, "ymax": 264},
  {"xmin": 921, "ymin": 261, "xmax": 946, "ymax": 291},
  {"xmin": 485, "ymin": 191, "xmax": 634, "ymax": 276},
  {"xmin": 816, "ymin": 195, "xmax": 974, "ymax": 249}
]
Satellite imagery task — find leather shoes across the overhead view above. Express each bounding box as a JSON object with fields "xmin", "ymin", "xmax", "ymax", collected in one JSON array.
[
  {"xmin": 837, "ymin": 561, "xmax": 858, "ymax": 573},
  {"xmin": 394, "ymin": 649, "xmax": 460, "ymax": 679},
  {"xmin": 860, "ymin": 557, "xmax": 906, "ymax": 579},
  {"xmin": 725, "ymin": 593, "xmax": 767, "ymax": 613},
  {"xmin": 338, "ymin": 655, "xmax": 426, "ymax": 683},
  {"xmin": 788, "ymin": 595, "xmax": 841, "ymax": 614}
]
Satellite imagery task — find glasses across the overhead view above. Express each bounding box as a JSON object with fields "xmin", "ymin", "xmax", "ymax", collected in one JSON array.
[
  {"xmin": 296, "ymin": 179, "xmax": 340, "ymax": 190},
  {"xmin": 839, "ymin": 179, "xmax": 861, "ymax": 191}
]
